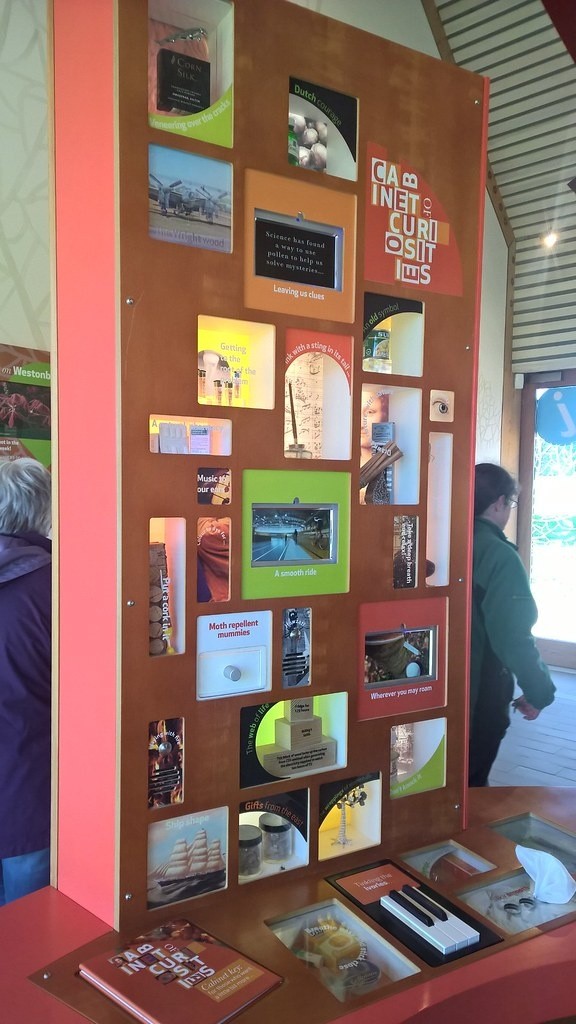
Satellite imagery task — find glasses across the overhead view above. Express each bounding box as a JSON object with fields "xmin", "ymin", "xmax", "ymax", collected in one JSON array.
[{"xmin": 504, "ymin": 495, "xmax": 519, "ymax": 508}]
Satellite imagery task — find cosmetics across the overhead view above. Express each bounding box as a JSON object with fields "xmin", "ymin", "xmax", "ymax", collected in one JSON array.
[{"xmin": 198, "ymin": 368, "xmax": 241, "ymax": 407}]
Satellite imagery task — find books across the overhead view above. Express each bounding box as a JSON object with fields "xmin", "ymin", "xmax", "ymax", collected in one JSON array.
[{"xmin": 78, "ymin": 920, "xmax": 284, "ymax": 1024}]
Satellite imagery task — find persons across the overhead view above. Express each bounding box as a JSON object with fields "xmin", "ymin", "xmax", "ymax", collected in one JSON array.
[
  {"xmin": 0, "ymin": 456, "xmax": 51, "ymax": 915},
  {"xmin": 467, "ymin": 463, "xmax": 558, "ymax": 786}
]
